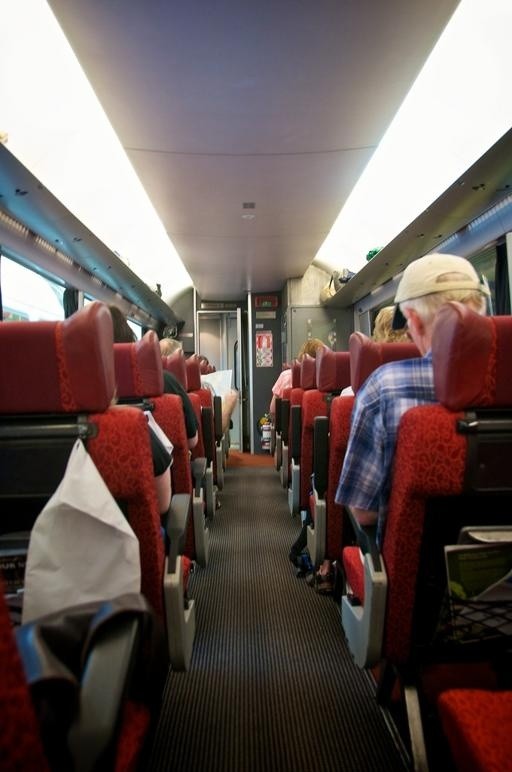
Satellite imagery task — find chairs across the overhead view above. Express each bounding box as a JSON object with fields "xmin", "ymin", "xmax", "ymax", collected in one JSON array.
[
  {"xmin": 270, "ymin": 301, "xmax": 512, "ymax": 772},
  {"xmin": 0, "ymin": 300, "xmax": 233, "ymax": 771}
]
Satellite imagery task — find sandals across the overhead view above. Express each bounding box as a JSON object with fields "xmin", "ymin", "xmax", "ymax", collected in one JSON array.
[{"xmin": 305, "ymin": 565, "xmax": 335, "ymax": 597}]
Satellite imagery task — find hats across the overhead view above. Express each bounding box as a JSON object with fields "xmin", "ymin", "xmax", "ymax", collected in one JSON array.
[{"xmin": 391, "ymin": 251, "xmax": 490, "ymax": 331}]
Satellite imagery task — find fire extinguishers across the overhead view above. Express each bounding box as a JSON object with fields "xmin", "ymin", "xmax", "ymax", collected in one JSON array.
[{"xmin": 257, "ymin": 413, "xmax": 272, "ymax": 450}]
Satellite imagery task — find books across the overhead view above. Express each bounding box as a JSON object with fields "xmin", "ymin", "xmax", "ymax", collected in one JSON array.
[{"xmin": 441, "ymin": 540, "xmax": 512, "ymax": 645}]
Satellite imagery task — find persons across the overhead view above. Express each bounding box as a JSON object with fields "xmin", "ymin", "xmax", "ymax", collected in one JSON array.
[
  {"xmin": 221, "ymin": 388, "xmax": 239, "ymax": 436},
  {"xmin": 269, "ymin": 338, "xmax": 326, "ymax": 418},
  {"xmin": 302, "ymin": 304, "xmax": 412, "ymax": 595},
  {"xmin": 109, "ymin": 302, "xmax": 198, "ymax": 452},
  {"xmin": 196, "ymin": 355, "xmax": 210, "ymax": 366},
  {"xmin": 142, "ymin": 408, "xmax": 177, "ymax": 525},
  {"xmin": 159, "ymin": 337, "xmax": 185, "ymax": 361},
  {"xmin": 329, "ymin": 252, "xmax": 488, "ymax": 529}
]
